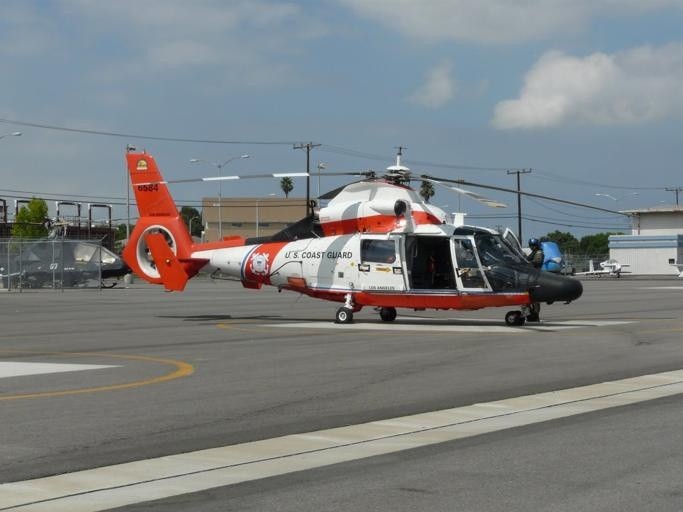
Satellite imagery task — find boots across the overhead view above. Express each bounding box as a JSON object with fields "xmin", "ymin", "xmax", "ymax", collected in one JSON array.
[
  {"xmin": 528, "ymin": 313, "xmax": 539, "ymax": 321},
  {"xmin": 527, "ymin": 312, "xmax": 533, "ymax": 318}
]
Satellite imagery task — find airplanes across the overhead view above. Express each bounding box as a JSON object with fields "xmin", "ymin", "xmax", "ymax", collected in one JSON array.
[
  {"xmin": 0, "ymin": 213, "xmax": 146, "ymax": 291},
  {"xmin": 571, "ymin": 254, "xmax": 636, "ymax": 277}
]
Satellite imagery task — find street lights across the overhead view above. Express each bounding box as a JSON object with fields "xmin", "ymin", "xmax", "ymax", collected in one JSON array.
[
  {"xmin": 315, "ymin": 160, "xmax": 328, "ymax": 210},
  {"xmin": 593, "ymin": 192, "xmax": 637, "ymax": 237},
  {"xmin": 186, "ymin": 154, "xmax": 251, "ymax": 241}
]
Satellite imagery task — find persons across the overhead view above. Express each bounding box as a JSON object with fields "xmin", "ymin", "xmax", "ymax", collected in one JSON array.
[{"xmin": 524, "ymin": 236, "xmax": 544, "ymax": 322}]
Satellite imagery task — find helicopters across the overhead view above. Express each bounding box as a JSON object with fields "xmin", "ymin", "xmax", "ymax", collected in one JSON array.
[{"xmin": 114, "ymin": 134, "xmax": 590, "ymax": 329}]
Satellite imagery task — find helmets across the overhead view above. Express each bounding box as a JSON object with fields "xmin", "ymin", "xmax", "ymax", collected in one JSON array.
[{"xmin": 529, "ymin": 238, "xmax": 538, "ymax": 245}]
